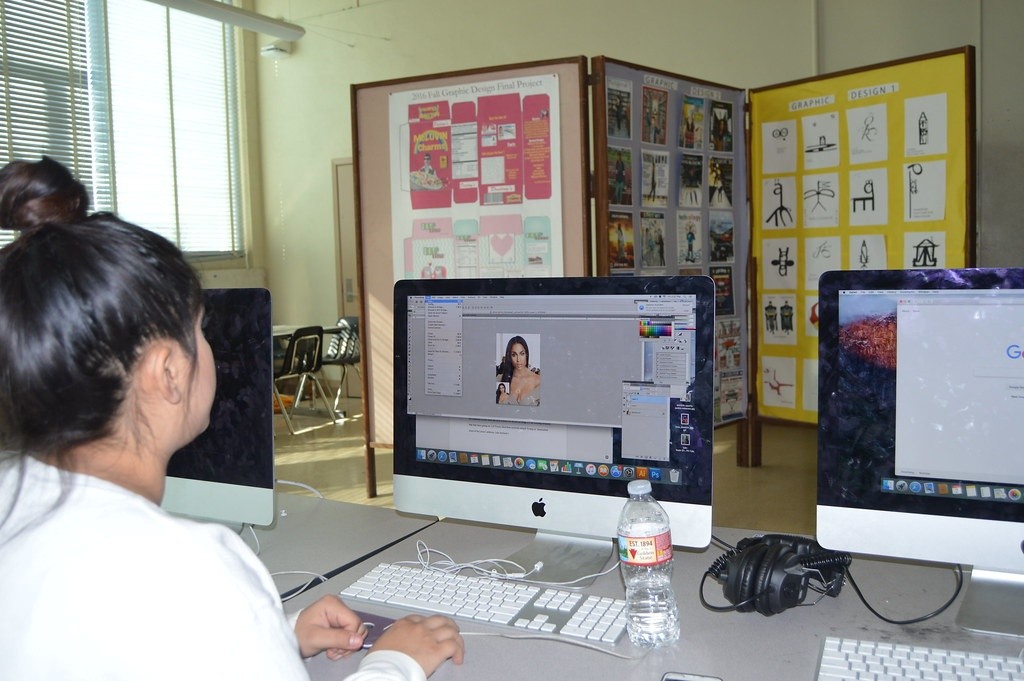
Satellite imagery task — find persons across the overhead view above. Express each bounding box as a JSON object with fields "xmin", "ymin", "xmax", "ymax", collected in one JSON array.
[
  {"xmin": 611, "ymin": 92, "xmax": 733, "ymax": 264},
  {"xmin": 495, "ymin": 335, "xmax": 540, "ymax": 406},
  {"xmin": 496, "ymin": 382, "xmax": 509, "ymax": 405},
  {"xmin": 0, "ymin": 155, "xmax": 464, "ymax": 681}
]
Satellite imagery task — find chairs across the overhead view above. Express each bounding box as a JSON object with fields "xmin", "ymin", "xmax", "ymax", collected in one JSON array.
[
  {"xmin": 273, "ymin": 326, "xmax": 337, "ymax": 438},
  {"xmin": 313, "ymin": 316, "xmax": 360, "ymax": 419}
]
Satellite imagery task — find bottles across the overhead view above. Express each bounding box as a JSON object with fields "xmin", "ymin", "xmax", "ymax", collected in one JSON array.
[{"xmin": 618, "ymin": 479, "xmax": 680, "ymax": 647}]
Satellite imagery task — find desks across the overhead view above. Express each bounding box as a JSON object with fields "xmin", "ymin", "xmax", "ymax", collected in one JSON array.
[
  {"xmin": 275, "ymin": 326, "xmax": 346, "ymax": 418},
  {"xmin": 238, "ymin": 490, "xmax": 1024, "ymax": 681}
]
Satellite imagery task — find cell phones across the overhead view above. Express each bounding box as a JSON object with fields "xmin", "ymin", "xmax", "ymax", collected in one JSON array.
[
  {"xmin": 349, "ymin": 607, "xmax": 397, "ymax": 647},
  {"xmin": 661, "ymin": 672, "xmax": 723, "ymax": 681}
]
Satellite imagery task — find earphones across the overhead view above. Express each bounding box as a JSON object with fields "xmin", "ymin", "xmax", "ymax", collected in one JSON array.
[{"xmin": 491, "ymin": 569, "xmax": 499, "ymax": 578}]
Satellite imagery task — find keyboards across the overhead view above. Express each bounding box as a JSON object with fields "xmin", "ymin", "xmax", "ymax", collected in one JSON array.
[
  {"xmin": 813, "ymin": 635, "xmax": 1024, "ymax": 681},
  {"xmin": 339, "ymin": 562, "xmax": 629, "ymax": 649}
]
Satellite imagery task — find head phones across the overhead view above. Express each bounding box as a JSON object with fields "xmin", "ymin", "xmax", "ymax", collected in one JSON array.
[{"xmin": 718, "ymin": 533, "xmax": 848, "ymax": 617}]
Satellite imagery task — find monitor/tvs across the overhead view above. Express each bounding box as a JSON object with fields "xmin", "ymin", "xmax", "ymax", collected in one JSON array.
[
  {"xmin": 158, "ymin": 288, "xmax": 273, "ymax": 535},
  {"xmin": 817, "ymin": 266, "xmax": 1024, "ymax": 637},
  {"xmin": 392, "ymin": 275, "xmax": 716, "ymax": 589}
]
